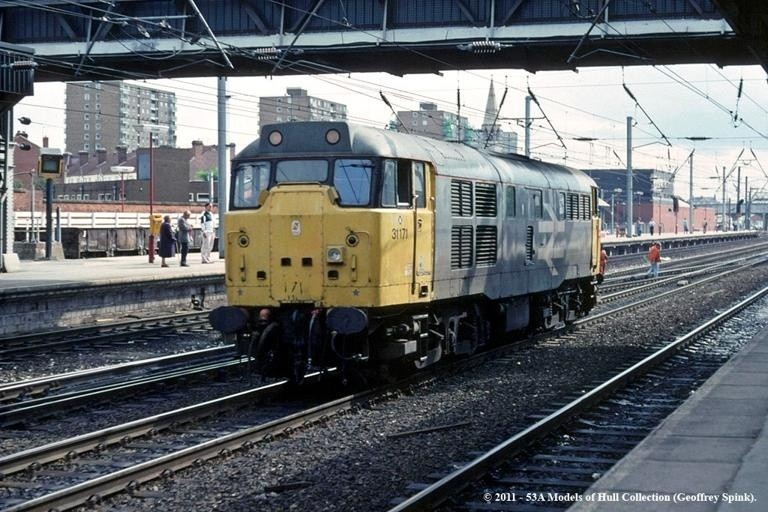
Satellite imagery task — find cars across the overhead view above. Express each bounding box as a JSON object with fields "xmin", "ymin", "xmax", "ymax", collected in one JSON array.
[{"xmin": 715, "ymin": 215, "xmax": 746, "ymax": 232}]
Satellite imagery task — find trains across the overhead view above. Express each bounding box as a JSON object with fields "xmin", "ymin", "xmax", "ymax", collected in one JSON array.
[{"xmin": 207, "ymin": 119, "xmax": 605, "ymax": 379}]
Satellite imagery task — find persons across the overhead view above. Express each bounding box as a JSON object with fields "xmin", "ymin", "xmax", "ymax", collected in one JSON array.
[
  {"xmin": 703, "ymin": 218, "xmax": 707, "ymax": 234},
  {"xmin": 200, "ymin": 202, "xmax": 216, "ymax": 264},
  {"xmin": 636, "ymin": 217, "xmax": 644, "ymax": 236},
  {"xmin": 645, "ymin": 241, "xmax": 661, "ymax": 277},
  {"xmin": 599, "ymin": 246, "xmax": 608, "ymax": 275},
  {"xmin": 178, "ymin": 210, "xmax": 193, "ymax": 267},
  {"xmin": 649, "ymin": 218, "xmax": 656, "ymax": 236},
  {"xmin": 683, "ymin": 218, "xmax": 688, "ymax": 234},
  {"xmin": 158, "ymin": 216, "xmax": 177, "ymax": 267}
]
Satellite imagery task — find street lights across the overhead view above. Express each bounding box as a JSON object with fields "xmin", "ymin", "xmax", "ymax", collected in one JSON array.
[
  {"xmin": 613, "ymin": 187, "xmax": 621, "ymax": 237},
  {"xmin": 635, "ymin": 191, "xmax": 645, "ymax": 222}
]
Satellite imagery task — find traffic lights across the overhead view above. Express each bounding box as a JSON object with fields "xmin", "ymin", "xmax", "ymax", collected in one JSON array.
[{"xmin": 39, "ymin": 154, "xmax": 61, "ymax": 176}]
